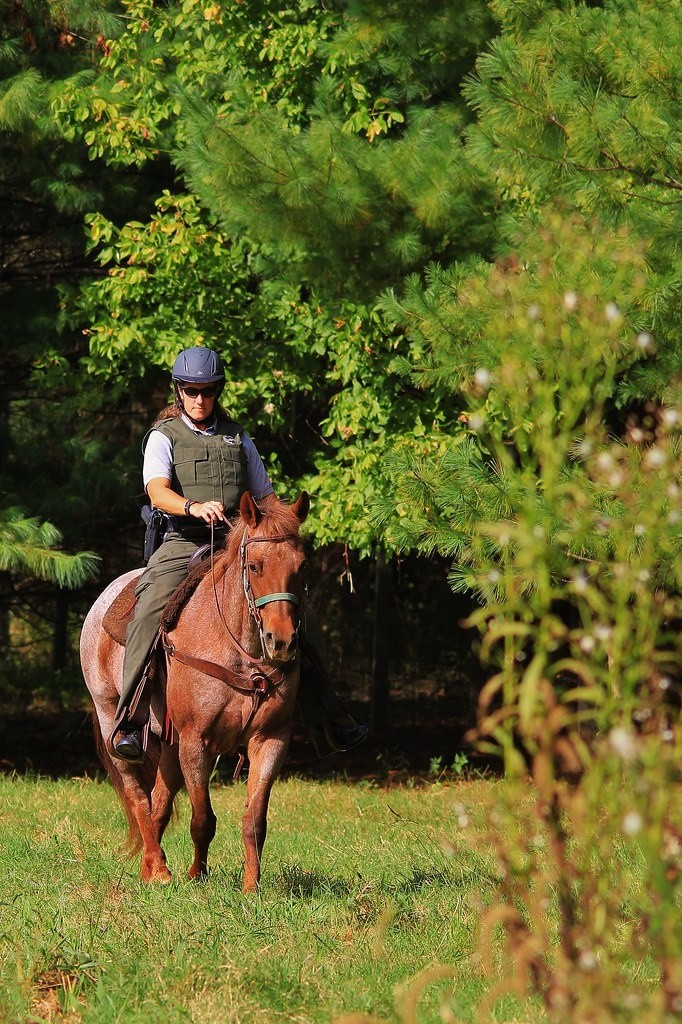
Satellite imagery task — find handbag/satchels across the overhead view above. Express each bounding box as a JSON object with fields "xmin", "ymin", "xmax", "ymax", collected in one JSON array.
[{"xmin": 141, "ymin": 506, "xmax": 166, "ymax": 560}]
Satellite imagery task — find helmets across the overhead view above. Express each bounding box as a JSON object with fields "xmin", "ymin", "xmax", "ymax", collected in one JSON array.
[{"xmin": 171, "ymin": 347, "xmax": 226, "ymax": 399}]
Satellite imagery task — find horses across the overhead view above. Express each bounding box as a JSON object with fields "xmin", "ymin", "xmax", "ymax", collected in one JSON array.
[{"xmin": 78, "ymin": 492, "xmax": 311, "ymax": 896}]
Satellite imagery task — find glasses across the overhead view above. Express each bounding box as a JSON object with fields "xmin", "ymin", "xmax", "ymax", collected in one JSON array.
[{"xmin": 179, "ymin": 384, "xmax": 220, "ymax": 398}]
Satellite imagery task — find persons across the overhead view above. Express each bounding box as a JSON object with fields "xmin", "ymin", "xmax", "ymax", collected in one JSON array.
[{"xmin": 117, "ymin": 347, "xmax": 280, "ymax": 760}]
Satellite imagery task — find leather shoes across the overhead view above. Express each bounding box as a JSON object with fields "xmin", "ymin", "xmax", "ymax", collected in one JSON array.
[{"xmin": 116, "ymin": 725, "xmax": 142, "ymax": 758}]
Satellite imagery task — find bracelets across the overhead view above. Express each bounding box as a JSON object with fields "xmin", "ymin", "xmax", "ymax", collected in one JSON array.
[{"xmin": 184, "ymin": 499, "xmax": 199, "ymax": 517}]
